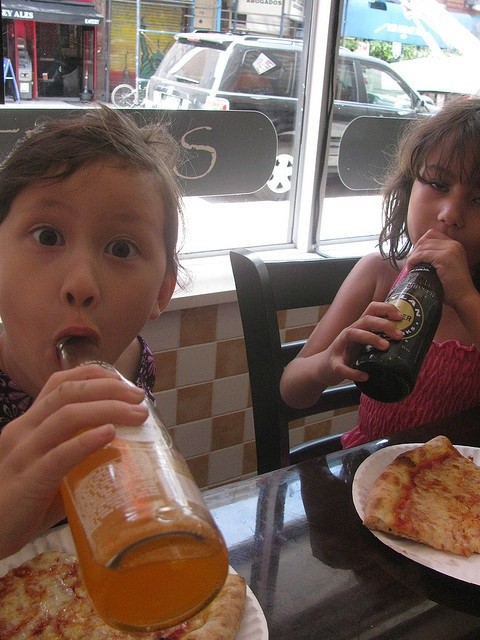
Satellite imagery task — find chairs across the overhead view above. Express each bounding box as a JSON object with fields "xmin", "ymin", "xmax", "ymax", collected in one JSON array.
[{"xmin": 230, "ymin": 248, "xmax": 362, "ymax": 475}]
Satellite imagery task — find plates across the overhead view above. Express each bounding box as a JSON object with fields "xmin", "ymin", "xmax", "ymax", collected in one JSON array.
[
  {"xmin": 350, "ymin": 441, "xmax": 479, "ymax": 586},
  {"xmin": 0, "ymin": 521, "xmax": 269, "ymax": 640}
]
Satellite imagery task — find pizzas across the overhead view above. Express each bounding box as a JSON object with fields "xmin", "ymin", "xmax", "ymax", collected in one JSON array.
[
  {"xmin": 363, "ymin": 435, "xmax": 480, "ymax": 558},
  {"xmin": 2, "ymin": 550, "xmax": 247, "ymax": 639}
]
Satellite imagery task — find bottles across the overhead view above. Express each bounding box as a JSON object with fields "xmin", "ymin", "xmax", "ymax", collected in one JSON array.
[
  {"xmin": 351, "ymin": 264, "xmax": 445, "ymax": 404},
  {"xmin": 54, "ymin": 334, "xmax": 230, "ymax": 632}
]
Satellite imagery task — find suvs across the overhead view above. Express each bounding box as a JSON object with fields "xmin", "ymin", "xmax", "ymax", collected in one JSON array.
[{"xmin": 147, "ymin": 25, "xmax": 454, "ymax": 199}]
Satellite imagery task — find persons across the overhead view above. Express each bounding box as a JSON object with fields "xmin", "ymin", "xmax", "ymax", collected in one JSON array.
[
  {"xmin": 0, "ymin": 105, "xmax": 189, "ymax": 563},
  {"xmin": 280, "ymin": 99, "xmax": 480, "ymax": 448}
]
